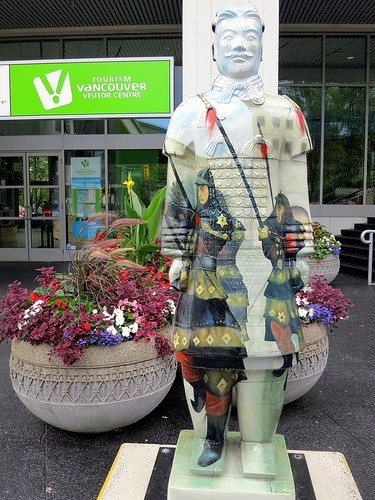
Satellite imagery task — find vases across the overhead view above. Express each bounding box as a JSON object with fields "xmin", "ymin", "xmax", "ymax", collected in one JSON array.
[
  {"xmin": 230, "ymin": 321, "xmax": 333, "ymax": 412},
  {"xmin": 7, "ymin": 325, "xmax": 178, "ymax": 432},
  {"xmin": 303, "ymin": 253, "xmax": 340, "ymax": 285}
]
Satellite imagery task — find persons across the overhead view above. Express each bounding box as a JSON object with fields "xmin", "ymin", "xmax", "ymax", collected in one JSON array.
[
  {"xmin": 256, "ymin": 191, "xmax": 307, "ymax": 377},
  {"xmin": 177, "ymin": 169, "xmax": 250, "ymax": 468},
  {"xmin": 158, "ymin": 5, "xmax": 315, "ymax": 481}
]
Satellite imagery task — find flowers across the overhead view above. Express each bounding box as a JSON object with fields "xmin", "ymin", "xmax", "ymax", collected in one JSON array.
[
  {"xmin": 303, "ymin": 221, "xmax": 343, "ymax": 259},
  {"xmin": 291, "ymin": 271, "xmax": 356, "ymax": 333},
  {"xmin": 1, "ymin": 258, "xmax": 182, "ymax": 368}
]
